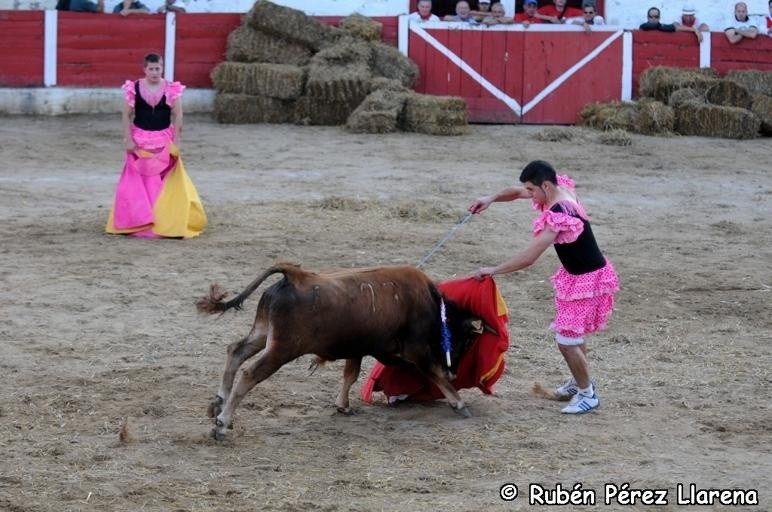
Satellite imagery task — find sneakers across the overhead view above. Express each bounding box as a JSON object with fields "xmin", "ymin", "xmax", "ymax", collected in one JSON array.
[
  {"xmin": 561, "ymin": 393, "xmax": 600, "ymax": 414},
  {"xmin": 556, "ymin": 382, "xmax": 595, "ymax": 397}
]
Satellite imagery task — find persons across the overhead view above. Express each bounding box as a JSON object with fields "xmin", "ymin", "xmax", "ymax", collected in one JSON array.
[
  {"xmin": 465, "ymin": 160, "xmax": 619, "ymax": 415},
  {"xmin": 409, "ymin": 0, "xmax": 772, "ymax": 46},
  {"xmin": 156, "ymin": 1, "xmax": 188, "ymax": 15},
  {"xmin": 69, "ymin": 1, "xmax": 106, "ymax": 14},
  {"xmin": 106, "ymin": 50, "xmax": 205, "ymax": 240},
  {"xmin": 112, "ymin": 1, "xmax": 151, "ymax": 17}
]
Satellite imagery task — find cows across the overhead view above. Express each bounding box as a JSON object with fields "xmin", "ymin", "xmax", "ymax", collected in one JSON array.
[{"xmin": 191, "ymin": 256, "xmax": 499, "ymax": 442}]
